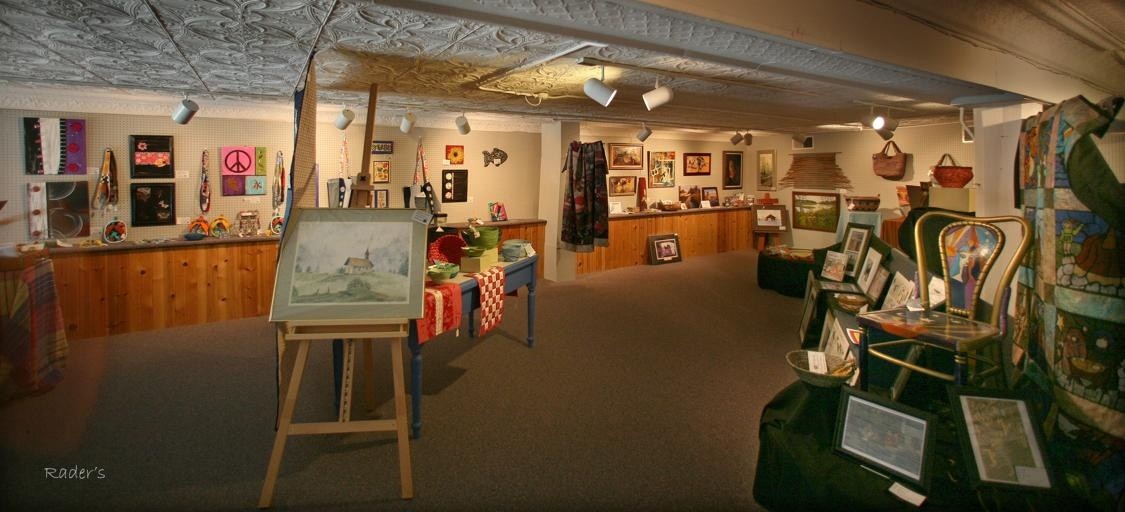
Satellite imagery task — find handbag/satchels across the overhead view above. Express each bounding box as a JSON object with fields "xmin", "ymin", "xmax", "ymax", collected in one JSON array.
[{"xmin": 872, "ymin": 141, "xmax": 907, "ymax": 180}]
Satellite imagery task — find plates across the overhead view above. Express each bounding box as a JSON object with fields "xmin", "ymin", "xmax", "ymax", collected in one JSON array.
[{"xmin": 184, "ymin": 234, "xmax": 207, "ymax": 240}]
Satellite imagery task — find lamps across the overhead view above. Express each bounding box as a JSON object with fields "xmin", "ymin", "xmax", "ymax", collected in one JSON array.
[
  {"xmin": 171, "ymin": 89, "xmax": 200, "ymax": 126},
  {"xmin": 572, "ymin": 56, "xmax": 674, "ymax": 114},
  {"xmin": 791, "ymin": 130, "xmax": 808, "ymax": 144},
  {"xmin": 867, "ymin": 101, "xmax": 902, "ymax": 142},
  {"xmin": 331, "ymin": 101, "xmax": 476, "ymax": 136},
  {"xmin": 635, "ymin": 122, "xmax": 653, "ymax": 142},
  {"xmin": 729, "ymin": 129, "xmax": 755, "ymax": 147}
]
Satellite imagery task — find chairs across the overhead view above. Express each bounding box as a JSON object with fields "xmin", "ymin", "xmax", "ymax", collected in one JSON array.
[{"xmin": 854, "ymin": 209, "xmax": 1033, "ymax": 406}]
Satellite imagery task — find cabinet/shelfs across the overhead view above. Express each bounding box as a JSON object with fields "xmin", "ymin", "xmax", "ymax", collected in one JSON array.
[
  {"xmin": 446, "ymin": 221, "xmax": 549, "ymax": 281},
  {"xmin": 574, "ymin": 204, "xmax": 788, "ymax": 279},
  {"xmin": 36, "ymin": 235, "xmax": 288, "ymax": 340}
]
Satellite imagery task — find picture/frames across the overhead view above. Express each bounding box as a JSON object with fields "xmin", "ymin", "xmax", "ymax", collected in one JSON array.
[
  {"xmin": 702, "ymin": 187, "xmax": 719, "ymax": 207},
  {"xmin": 828, "ymin": 381, "xmax": 941, "ymax": 497},
  {"xmin": 751, "ymin": 203, "xmax": 789, "ymax": 233},
  {"xmin": 267, "ymin": 205, "xmax": 431, "ymax": 323},
  {"xmin": 129, "ymin": 181, "xmax": 177, "ymax": 228},
  {"xmin": 790, "ymin": 219, "xmax": 915, "ymax": 389},
  {"xmin": 790, "ymin": 190, "xmax": 841, "ymax": 234},
  {"xmin": 646, "ymin": 233, "xmax": 683, "ymax": 266},
  {"xmin": 608, "ymin": 175, "xmax": 637, "ymax": 196},
  {"xmin": 755, "ymin": 147, "xmax": 778, "ymax": 192},
  {"xmin": 127, "ymin": 133, "xmax": 175, "ymax": 180},
  {"xmin": 946, "ymin": 384, "xmax": 1058, "ymax": 497},
  {"xmin": 682, "ymin": 152, "xmax": 712, "ymax": 176},
  {"xmin": 721, "ymin": 150, "xmax": 743, "ymax": 190},
  {"xmin": 606, "ymin": 142, "xmax": 644, "ymax": 171}
]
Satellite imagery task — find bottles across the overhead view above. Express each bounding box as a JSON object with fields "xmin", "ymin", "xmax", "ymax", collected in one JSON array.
[{"xmin": 238, "ymin": 218, "xmax": 257, "ymax": 237}]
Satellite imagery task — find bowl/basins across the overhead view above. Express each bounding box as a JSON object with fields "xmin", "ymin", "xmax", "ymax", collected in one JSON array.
[
  {"xmin": 429, "ymin": 263, "xmax": 460, "ymax": 284},
  {"xmin": 461, "ymin": 247, "xmax": 484, "ymax": 256},
  {"xmin": 934, "ymin": 166, "xmax": 973, "ymax": 187},
  {"xmin": 846, "ymin": 197, "xmax": 881, "ymax": 212}
]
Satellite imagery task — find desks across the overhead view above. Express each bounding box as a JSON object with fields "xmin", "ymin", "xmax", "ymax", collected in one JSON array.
[
  {"xmin": 752, "ymin": 344, "xmax": 1111, "ymax": 512},
  {"xmin": 756, "ymin": 240, "xmax": 841, "ymax": 300},
  {"xmin": 0, "ymin": 257, "xmax": 72, "ymax": 405},
  {"xmin": 332, "ymin": 251, "xmax": 537, "ymax": 440}
]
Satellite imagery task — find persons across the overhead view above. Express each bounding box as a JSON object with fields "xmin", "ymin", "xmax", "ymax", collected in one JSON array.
[{"xmin": 726, "ymin": 160, "xmax": 740, "ymax": 186}]
